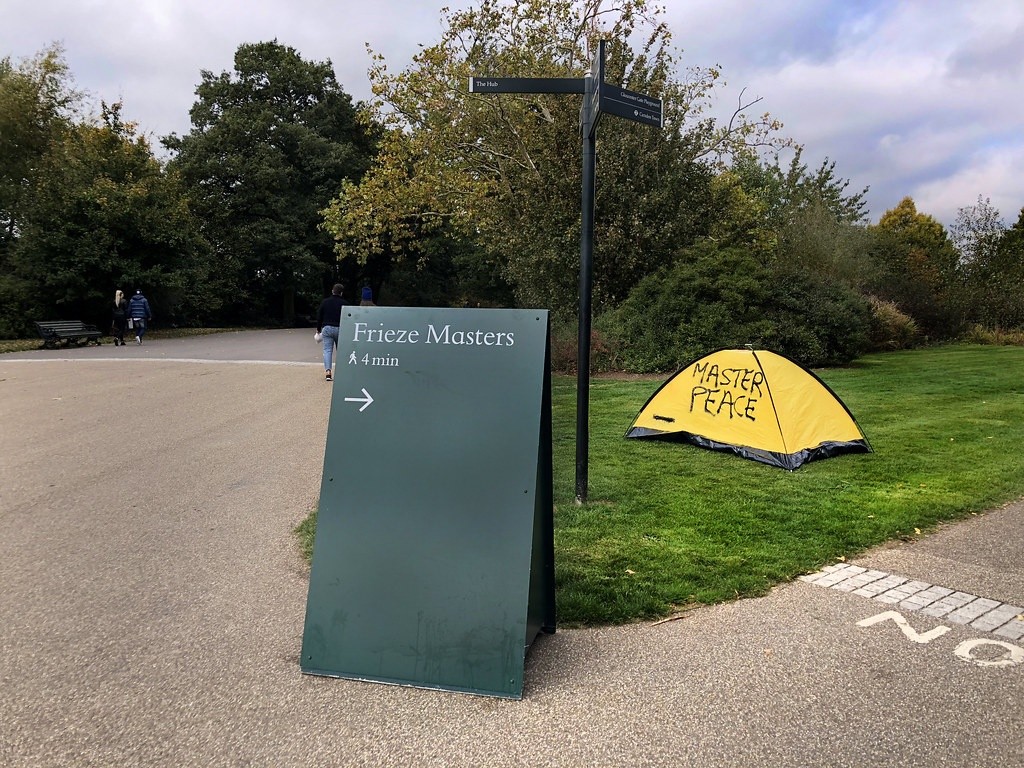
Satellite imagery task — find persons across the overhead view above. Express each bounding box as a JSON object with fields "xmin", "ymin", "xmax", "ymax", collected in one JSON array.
[
  {"xmin": 360, "ymin": 287, "xmax": 376, "ymax": 306},
  {"xmin": 317, "ymin": 284, "xmax": 353, "ymax": 381},
  {"xmin": 112, "ymin": 289, "xmax": 152, "ymax": 346}
]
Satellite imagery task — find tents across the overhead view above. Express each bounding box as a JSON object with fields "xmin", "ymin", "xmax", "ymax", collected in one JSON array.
[{"xmin": 622, "ymin": 343, "xmax": 876, "ymax": 473}]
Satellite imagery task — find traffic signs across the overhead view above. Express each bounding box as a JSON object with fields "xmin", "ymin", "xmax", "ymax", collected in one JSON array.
[
  {"xmin": 604, "ymin": 82, "xmax": 664, "ymax": 128},
  {"xmin": 586, "ymin": 38, "xmax": 606, "ymax": 139},
  {"xmin": 468, "ymin": 75, "xmax": 586, "ymax": 94}
]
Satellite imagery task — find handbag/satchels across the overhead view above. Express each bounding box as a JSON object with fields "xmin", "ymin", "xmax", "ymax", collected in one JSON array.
[{"xmin": 112, "ymin": 320, "xmax": 116, "ymax": 328}]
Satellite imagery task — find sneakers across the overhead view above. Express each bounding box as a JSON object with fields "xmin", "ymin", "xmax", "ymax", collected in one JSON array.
[{"xmin": 326, "ymin": 374, "xmax": 331, "ymax": 381}]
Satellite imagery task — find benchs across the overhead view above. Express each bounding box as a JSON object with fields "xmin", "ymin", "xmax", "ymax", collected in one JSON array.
[{"xmin": 33, "ymin": 320, "xmax": 102, "ymax": 350}]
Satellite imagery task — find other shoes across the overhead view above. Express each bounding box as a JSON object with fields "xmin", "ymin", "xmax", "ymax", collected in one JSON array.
[
  {"xmin": 113, "ymin": 338, "xmax": 118, "ymax": 346},
  {"xmin": 121, "ymin": 342, "xmax": 126, "ymax": 345},
  {"xmin": 136, "ymin": 336, "xmax": 140, "ymax": 344}
]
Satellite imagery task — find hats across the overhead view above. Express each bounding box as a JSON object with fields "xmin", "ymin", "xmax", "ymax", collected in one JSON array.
[
  {"xmin": 136, "ymin": 288, "xmax": 141, "ymax": 290},
  {"xmin": 362, "ymin": 287, "xmax": 372, "ymax": 301}
]
